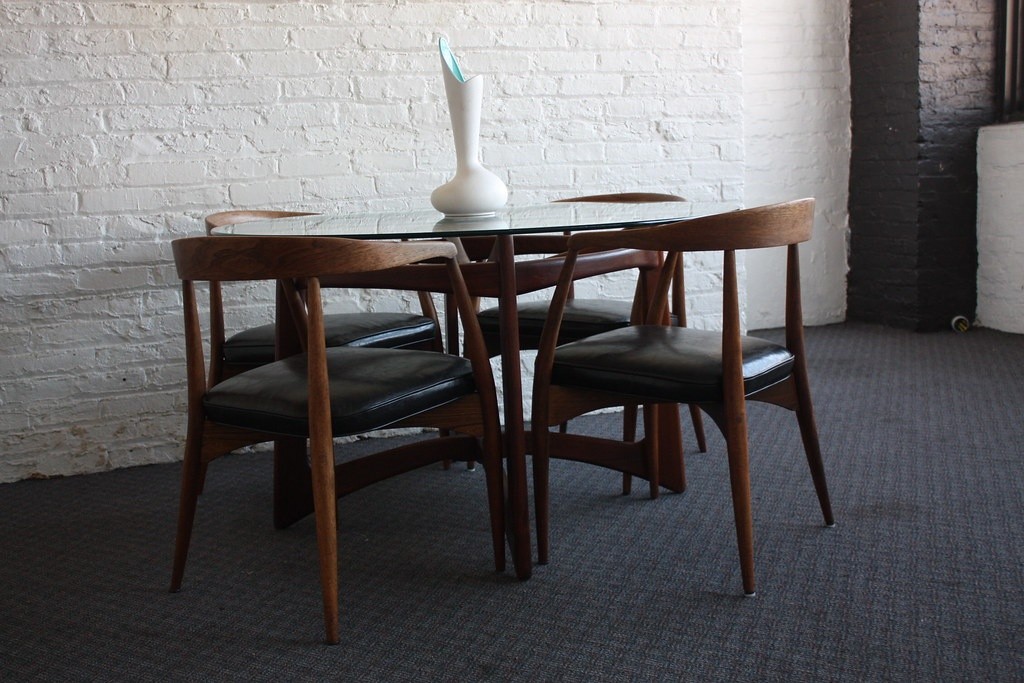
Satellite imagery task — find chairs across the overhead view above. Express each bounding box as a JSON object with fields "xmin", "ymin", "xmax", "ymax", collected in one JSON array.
[
  {"xmin": 530, "ymin": 198, "xmax": 839, "ymax": 601},
  {"xmin": 205, "ymin": 208, "xmax": 458, "ymax": 521},
  {"xmin": 464, "ymin": 191, "xmax": 709, "ymax": 495},
  {"xmin": 167, "ymin": 234, "xmax": 508, "ymax": 645}
]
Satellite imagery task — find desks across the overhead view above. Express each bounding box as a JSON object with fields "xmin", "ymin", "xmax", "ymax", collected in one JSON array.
[{"xmin": 210, "ymin": 200, "xmax": 740, "ymax": 582}]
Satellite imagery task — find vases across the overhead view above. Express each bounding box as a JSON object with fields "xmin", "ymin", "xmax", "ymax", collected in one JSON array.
[{"xmin": 430, "ymin": 38, "xmax": 508, "ymax": 220}]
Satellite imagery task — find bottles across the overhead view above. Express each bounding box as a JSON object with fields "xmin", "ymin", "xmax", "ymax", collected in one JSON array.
[{"xmin": 431, "ymin": 36, "xmax": 507, "ymax": 216}]
